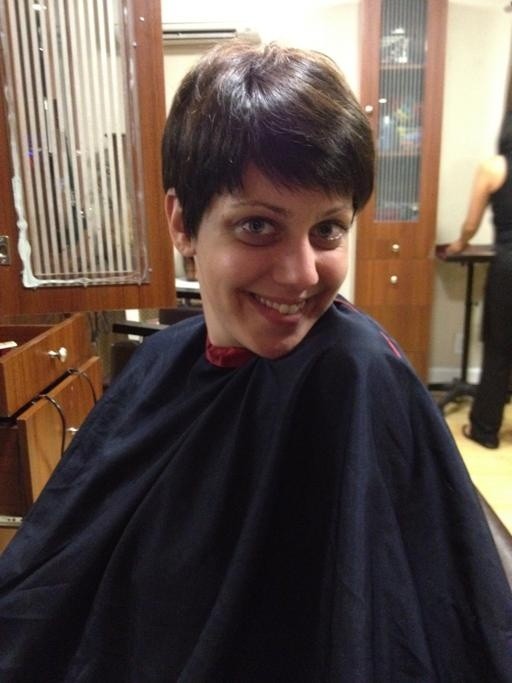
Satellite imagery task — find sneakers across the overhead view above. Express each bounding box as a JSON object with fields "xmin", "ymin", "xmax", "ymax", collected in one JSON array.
[{"xmin": 461, "ymin": 422, "xmax": 500, "ymax": 451}]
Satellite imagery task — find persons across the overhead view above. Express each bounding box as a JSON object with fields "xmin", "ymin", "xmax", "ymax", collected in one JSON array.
[
  {"xmin": 449, "ymin": 155, "xmax": 512, "ymax": 449},
  {"xmin": 1, "ymin": 30, "xmax": 509, "ymax": 678}
]
